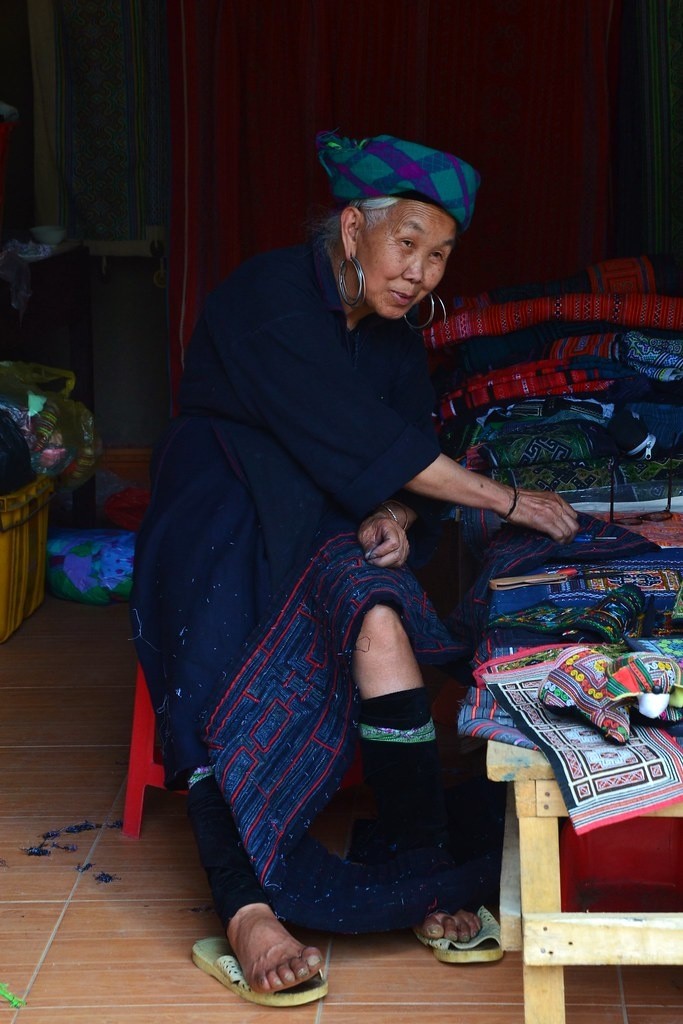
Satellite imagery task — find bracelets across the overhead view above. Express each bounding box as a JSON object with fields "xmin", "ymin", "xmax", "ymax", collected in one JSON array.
[
  {"xmin": 500, "ymin": 486, "xmax": 517, "ymax": 520},
  {"xmin": 382, "ymin": 501, "xmax": 408, "ymax": 530}
]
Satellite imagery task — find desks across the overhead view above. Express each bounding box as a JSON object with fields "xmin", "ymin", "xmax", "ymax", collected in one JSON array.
[{"xmin": 486, "ymin": 740, "xmax": 683, "ymax": 1024}]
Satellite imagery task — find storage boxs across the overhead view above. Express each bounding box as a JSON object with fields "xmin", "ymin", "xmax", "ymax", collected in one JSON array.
[
  {"xmin": 96, "ymin": 446, "xmax": 154, "ymax": 517},
  {"xmin": 1, "ymin": 474, "xmax": 58, "ymax": 643}
]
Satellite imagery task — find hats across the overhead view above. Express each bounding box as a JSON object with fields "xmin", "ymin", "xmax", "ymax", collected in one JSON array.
[{"xmin": 316, "ymin": 126, "xmax": 481, "ymax": 229}]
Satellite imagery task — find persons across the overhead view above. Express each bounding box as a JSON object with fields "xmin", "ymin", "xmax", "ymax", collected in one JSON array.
[{"xmin": 129, "ymin": 136, "xmax": 579, "ymax": 993}]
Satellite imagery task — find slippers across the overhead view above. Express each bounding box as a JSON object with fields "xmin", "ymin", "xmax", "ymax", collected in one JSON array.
[
  {"xmin": 415, "ymin": 906, "xmax": 503, "ymax": 964},
  {"xmin": 192, "ymin": 937, "xmax": 329, "ymax": 1008}
]
